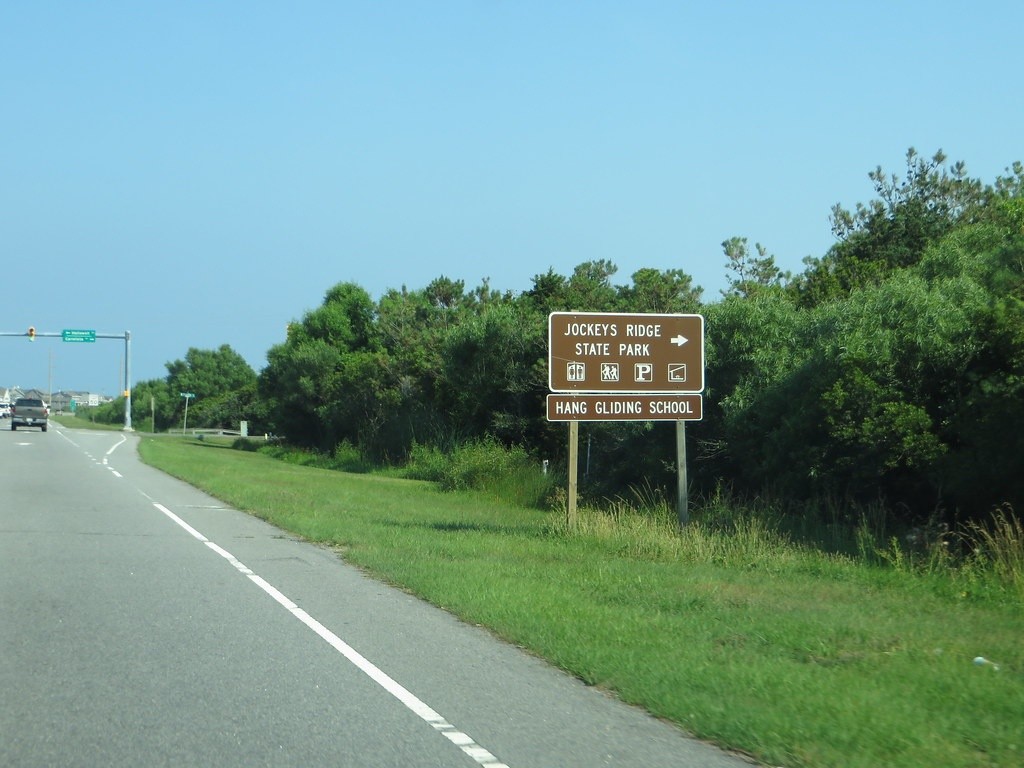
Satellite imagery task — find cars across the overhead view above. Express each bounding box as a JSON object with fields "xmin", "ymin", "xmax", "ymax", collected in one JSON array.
[
  {"xmin": 9, "ymin": 396, "xmax": 52, "ymax": 436},
  {"xmin": 0, "ymin": 403, "xmax": 12, "ymax": 420}
]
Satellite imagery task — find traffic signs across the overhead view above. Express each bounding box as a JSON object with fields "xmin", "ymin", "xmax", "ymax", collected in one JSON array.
[{"xmin": 547, "ymin": 309, "xmax": 706, "ymax": 395}]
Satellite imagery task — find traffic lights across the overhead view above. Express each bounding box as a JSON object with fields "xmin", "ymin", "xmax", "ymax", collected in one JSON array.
[{"xmin": 29, "ymin": 327, "xmax": 35, "ymax": 341}]
